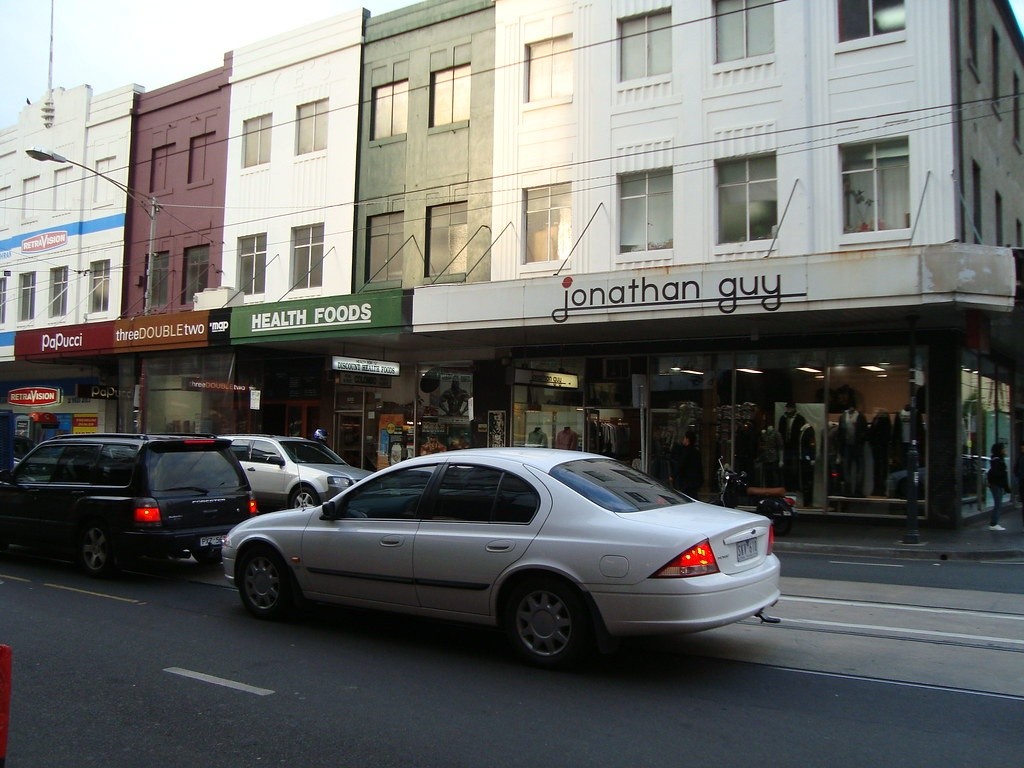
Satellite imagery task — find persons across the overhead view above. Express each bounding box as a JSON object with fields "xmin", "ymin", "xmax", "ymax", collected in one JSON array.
[
  {"xmin": 420, "ymin": 433, "xmax": 447, "ymax": 456},
  {"xmin": 670, "ymin": 431, "xmax": 705, "ymax": 500},
  {"xmin": 836, "ymin": 403, "xmax": 925, "ymax": 493},
  {"xmin": 439, "ymin": 376, "xmax": 471, "ymax": 417},
  {"xmin": 987, "ymin": 443, "xmax": 1011, "ymax": 531},
  {"xmin": 314, "ymin": 429, "xmax": 330, "ymax": 443},
  {"xmin": 527, "ymin": 427, "xmax": 548, "ymax": 448},
  {"xmin": 759, "ymin": 426, "xmax": 784, "ymax": 488},
  {"xmin": 777, "ymin": 401, "xmax": 806, "ymax": 474},
  {"xmin": 555, "ymin": 427, "xmax": 578, "ymax": 450},
  {"xmin": 798, "ymin": 423, "xmax": 815, "ymax": 507},
  {"xmin": 1013, "ymin": 441, "xmax": 1024, "ymax": 524}
]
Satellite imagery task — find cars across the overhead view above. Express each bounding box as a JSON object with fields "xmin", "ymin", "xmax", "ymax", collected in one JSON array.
[
  {"xmin": 10, "ymin": 436, "xmax": 37, "ymax": 472},
  {"xmin": 886, "ymin": 454, "xmax": 1009, "ymax": 504},
  {"xmin": 221, "ymin": 446, "xmax": 784, "ymax": 667}
]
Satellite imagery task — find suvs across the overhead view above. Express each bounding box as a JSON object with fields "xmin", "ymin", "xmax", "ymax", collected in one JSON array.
[
  {"xmin": 208, "ymin": 433, "xmax": 381, "ymax": 512},
  {"xmin": 0, "ymin": 431, "xmax": 261, "ymax": 577}
]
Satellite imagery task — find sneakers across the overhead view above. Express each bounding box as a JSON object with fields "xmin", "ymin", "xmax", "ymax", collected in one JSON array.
[{"xmin": 989, "ymin": 525, "xmax": 1006, "ymax": 531}]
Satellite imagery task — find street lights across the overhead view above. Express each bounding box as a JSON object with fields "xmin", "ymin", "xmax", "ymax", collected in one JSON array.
[{"xmin": 27, "ymin": 149, "xmax": 159, "ymax": 314}]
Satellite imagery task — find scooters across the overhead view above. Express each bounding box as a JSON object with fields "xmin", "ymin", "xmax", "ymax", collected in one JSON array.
[{"xmin": 707, "ymin": 453, "xmax": 799, "ymax": 534}]
